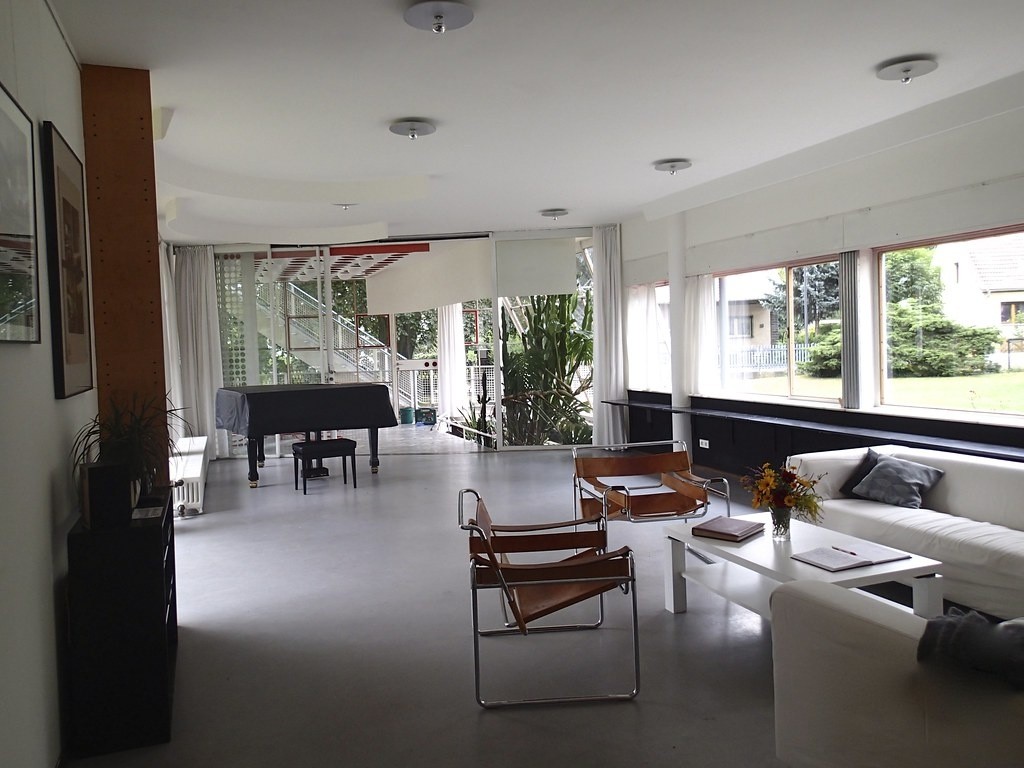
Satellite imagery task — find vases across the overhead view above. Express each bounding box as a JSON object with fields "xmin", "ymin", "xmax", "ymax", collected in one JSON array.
[{"xmin": 769, "ymin": 505, "xmax": 792, "ymax": 540}]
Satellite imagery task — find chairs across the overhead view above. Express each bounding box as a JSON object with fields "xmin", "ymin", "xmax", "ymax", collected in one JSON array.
[
  {"xmin": 571, "ymin": 438, "xmax": 731, "ymax": 555},
  {"xmin": 457, "ymin": 489, "xmax": 641, "ymax": 707}
]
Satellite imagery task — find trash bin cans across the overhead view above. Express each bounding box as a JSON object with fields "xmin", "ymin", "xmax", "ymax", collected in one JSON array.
[{"xmin": 400, "ymin": 407, "xmax": 414, "ymax": 424}]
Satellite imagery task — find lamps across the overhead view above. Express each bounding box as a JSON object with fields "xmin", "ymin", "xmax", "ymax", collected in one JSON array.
[
  {"xmin": 404, "ymin": 0, "xmax": 474, "ymax": 34},
  {"xmin": 333, "ymin": 201, "xmax": 358, "ymax": 210},
  {"xmin": 389, "ymin": 120, "xmax": 436, "ymax": 138},
  {"xmin": 655, "ymin": 160, "xmax": 690, "ymax": 175},
  {"xmin": 877, "ymin": 59, "xmax": 940, "ymax": 84},
  {"xmin": 540, "ymin": 210, "xmax": 568, "ymax": 221}
]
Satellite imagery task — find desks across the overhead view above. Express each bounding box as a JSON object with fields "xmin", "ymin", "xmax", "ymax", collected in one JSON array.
[{"xmin": 659, "ymin": 512, "xmax": 944, "ymax": 626}]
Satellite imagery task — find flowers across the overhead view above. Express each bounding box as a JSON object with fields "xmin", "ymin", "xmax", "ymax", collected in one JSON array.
[{"xmin": 739, "ymin": 460, "xmax": 829, "ymax": 524}]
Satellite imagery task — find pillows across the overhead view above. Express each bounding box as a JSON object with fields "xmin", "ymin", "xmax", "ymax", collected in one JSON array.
[
  {"xmin": 839, "ymin": 449, "xmax": 880, "ymax": 500},
  {"xmin": 852, "ymin": 454, "xmax": 945, "ymax": 508}
]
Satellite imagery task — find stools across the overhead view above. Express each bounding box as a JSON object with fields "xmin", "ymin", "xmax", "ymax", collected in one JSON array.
[{"xmin": 293, "ymin": 438, "xmax": 358, "ymax": 495}]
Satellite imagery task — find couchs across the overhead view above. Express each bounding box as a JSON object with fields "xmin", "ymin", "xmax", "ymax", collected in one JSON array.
[{"xmin": 785, "ymin": 443, "xmax": 1024, "ymax": 620}]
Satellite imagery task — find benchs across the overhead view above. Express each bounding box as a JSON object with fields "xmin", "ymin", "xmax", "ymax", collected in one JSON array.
[{"xmin": 169, "ymin": 436, "xmax": 209, "ymax": 516}]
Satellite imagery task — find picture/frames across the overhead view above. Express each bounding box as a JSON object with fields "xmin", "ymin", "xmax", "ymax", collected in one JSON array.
[
  {"xmin": 43, "ymin": 120, "xmax": 96, "ymax": 398},
  {"xmin": 0, "ymin": 83, "xmax": 41, "ymax": 344}
]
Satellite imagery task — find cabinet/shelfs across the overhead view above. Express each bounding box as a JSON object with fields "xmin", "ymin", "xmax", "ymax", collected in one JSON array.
[{"xmin": 67, "ymin": 489, "xmax": 179, "ymax": 751}]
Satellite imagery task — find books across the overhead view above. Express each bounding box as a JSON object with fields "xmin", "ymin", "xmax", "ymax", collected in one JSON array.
[
  {"xmin": 692, "ymin": 515, "xmax": 766, "ymax": 543},
  {"xmin": 790, "ymin": 541, "xmax": 913, "ymax": 573}
]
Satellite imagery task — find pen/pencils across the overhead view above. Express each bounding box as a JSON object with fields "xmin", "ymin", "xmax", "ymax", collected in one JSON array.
[{"xmin": 832, "ymin": 545, "xmax": 856, "ymax": 556}]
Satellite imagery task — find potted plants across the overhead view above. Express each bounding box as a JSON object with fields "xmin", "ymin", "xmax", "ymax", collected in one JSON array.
[{"xmin": 69, "ymin": 386, "xmax": 193, "ymax": 493}]
